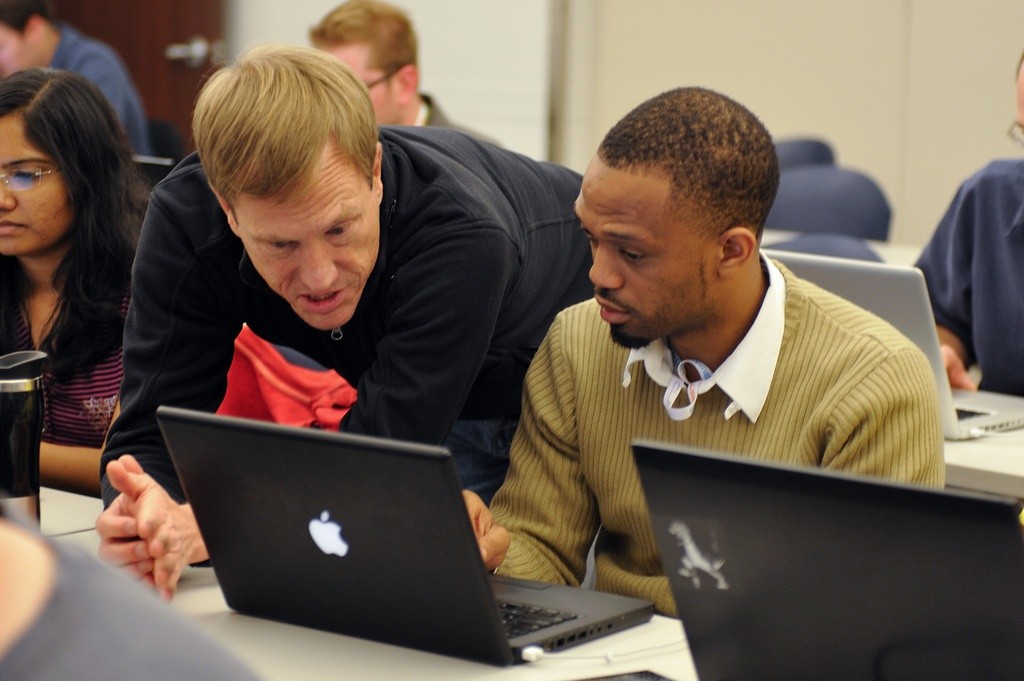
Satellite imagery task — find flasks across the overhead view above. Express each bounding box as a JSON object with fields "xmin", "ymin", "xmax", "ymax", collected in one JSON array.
[{"xmin": 0, "ymin": 351, "xmax": 50, "ymax": 535}]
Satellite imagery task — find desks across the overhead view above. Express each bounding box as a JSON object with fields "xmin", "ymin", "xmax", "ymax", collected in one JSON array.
[{"xmin": 38, "ymin": 230, "xmax": 1024, "ymax": 681}]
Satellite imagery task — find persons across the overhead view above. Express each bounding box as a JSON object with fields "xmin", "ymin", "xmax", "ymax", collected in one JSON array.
[
  {"xmin": 95, "ymin": 41, "xmax": 596, "ymax": 596},
  {"xmin": 915, "ymin": 52, "xmax": 1024, "ymax": 397},
  {"xmin": 0, "ymin": 0, "xmax": 496, "ymax": 504},
  {"xmin": 458, "ymin": 85, "xmax": 946, "ymax": 619}
]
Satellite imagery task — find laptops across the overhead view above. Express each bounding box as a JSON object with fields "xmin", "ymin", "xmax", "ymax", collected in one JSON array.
[
  {"xmin": 630, "ymin": 439, "xmax": 1024, "ymax": 681},
  {"xmin": 155, "ymin": 406, "xmax": 655, "ymax": 668},
  {"xmin": 759, "ymin": 248, "xmax": 1024, "ymax": 441}
]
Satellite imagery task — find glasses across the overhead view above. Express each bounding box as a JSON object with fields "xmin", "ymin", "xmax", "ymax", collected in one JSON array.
[
  {"xmin": 0, "ymin": 164, "xmax": 59, "ymax": 192},
  {"xmin": 1007, "ymin": 122, "xmax": 1024, "ymax": 143}
]
